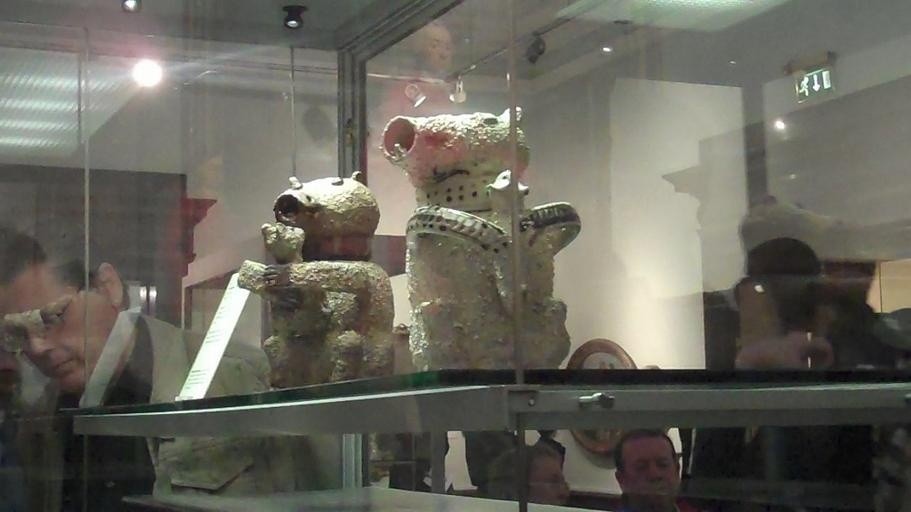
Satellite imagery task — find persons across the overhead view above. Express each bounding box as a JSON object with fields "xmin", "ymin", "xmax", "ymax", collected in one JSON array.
[
  {"xmin": 704, "ymin": 237, "xmax": 911, "ymax": 372},
  {"xmin": 0, "ymin": 217, "xmax": 342, "ymax": 512},
  {"xmin": 613, "ymin": 429, "xmax": 699, "ymax": 512},
  {"xmin": 488, "ymin": 447, "xmax": 570, "ymax": 505}
]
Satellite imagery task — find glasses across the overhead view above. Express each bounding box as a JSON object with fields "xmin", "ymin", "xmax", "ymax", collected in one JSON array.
[{"xmin": 0, "ymin": 282, "xmax": 86, "ymax": 353}]
